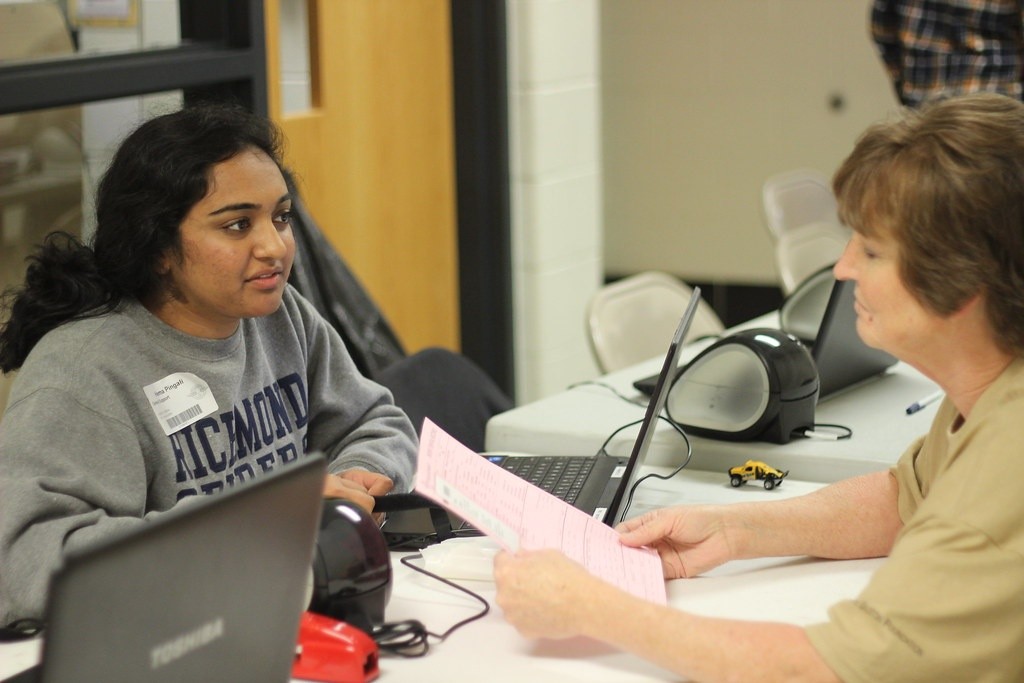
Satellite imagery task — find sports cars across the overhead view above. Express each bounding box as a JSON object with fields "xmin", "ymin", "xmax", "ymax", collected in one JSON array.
[{"xmin": 727, "ymin": 461, "xmax": 790, "ymax": 490}]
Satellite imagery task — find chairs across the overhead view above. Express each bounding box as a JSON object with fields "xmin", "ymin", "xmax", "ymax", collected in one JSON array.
[
  {"xmin": 366, "ymin": 342, "xmax": 515, "ymax": 456},
  {"xmin": 589, "ymin": 271, "xmax": 728, "ymax": 380},
  {"xmin": 765, "ymin": 170, "xmax": 853, "ymax": 298}
]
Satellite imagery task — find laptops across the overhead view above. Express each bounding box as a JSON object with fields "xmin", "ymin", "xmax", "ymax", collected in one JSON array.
[
  {"xmin": 634, "ymin": 278, "xmax": 900, "ymax": 403},
  {"xmin": 377, "ymin": 286, "xmax": 703, "ymax": 554},
  {"xmin": 0, "ymin": 452, "xmax": 328, "ymax": 683}
]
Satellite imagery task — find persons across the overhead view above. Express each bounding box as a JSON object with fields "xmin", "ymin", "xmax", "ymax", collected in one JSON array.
[
  {"xmin": 492, "ymin": 89, "xmax": 1024, "ymax": 683},
  {"xmin": 0, "ymin": 103, "xmax": 419, "ymax": 633}
]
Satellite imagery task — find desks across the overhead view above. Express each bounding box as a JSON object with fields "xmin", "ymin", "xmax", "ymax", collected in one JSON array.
[{"xmin": 281, "ymin": 307, "xmax": 949, "ymax": 683}]
